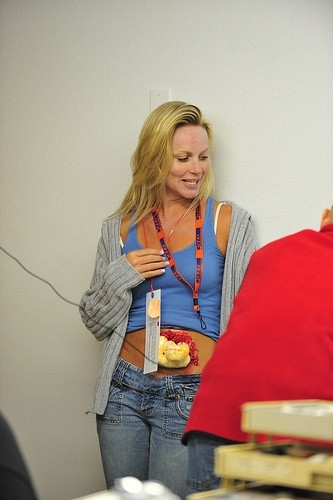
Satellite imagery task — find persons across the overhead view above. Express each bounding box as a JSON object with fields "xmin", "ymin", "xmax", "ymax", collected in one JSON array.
[
  {"xmin": 78, "ymin": 101, "xmax": 258, "ymax": 500},
  {"xmin": 182, "ymin": 205, "xmax": 332, "ymax": 492}
]
